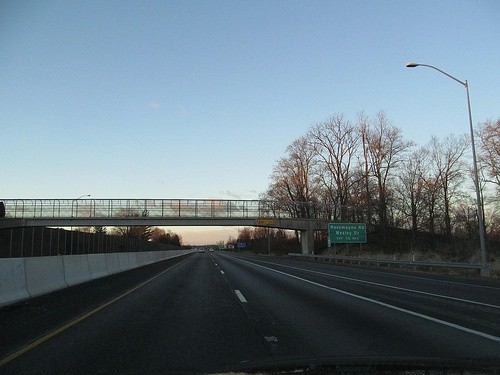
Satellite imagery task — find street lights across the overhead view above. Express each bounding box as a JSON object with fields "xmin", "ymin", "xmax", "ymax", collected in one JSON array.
[{"xmin": 408, "ymin": 62, "xmax": 488, "ymax": 263}]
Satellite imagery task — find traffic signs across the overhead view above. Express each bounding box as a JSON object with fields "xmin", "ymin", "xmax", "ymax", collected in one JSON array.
[{"xmin": 328, "ymin": 222, "xmax": 367, "ymax": 244}]
